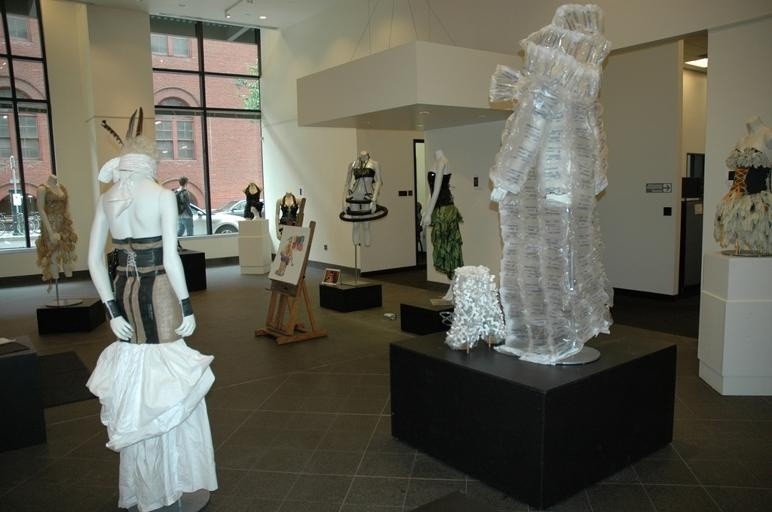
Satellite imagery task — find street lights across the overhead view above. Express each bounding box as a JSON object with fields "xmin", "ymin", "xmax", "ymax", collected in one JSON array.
[{"xmin": 9, "ymin": 155, "xmax": 19, "ymax": 212}]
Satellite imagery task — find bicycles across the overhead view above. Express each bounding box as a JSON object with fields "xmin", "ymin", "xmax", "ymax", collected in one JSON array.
[{"xmin": 0, "ymin": 212, "xmax": 42, "ymax": 237}]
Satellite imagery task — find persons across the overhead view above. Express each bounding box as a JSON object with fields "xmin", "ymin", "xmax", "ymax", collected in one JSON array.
[
  {"xmin": 418, "ymin": 150, "xmax": 463, "ymax": 301},
  {"xmin": 35, "ymin": 175, "xmax": 79, "ymax": 281},
  {"xmin": 86, "ymin": 136, "xmax": 219, "ymax": 511},
  {"xmin": 242, "ymin": 183, "xmax": 264, "ymax": 219},
  {"xmin": 275, "ymin": 191, "xmax": 301, "ymax": 239},
  {"xmin": 711, "ymin": 115, "xmax": 772, "ymax": 254},
  {"xmin": 176, "ymin": 176, "xmax": 195, "ymax": 237}
]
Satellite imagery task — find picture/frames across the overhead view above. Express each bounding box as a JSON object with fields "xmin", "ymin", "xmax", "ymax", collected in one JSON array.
[{"xmin": 321, "ymin": 268, "xmax": 341, "ymax": 285}]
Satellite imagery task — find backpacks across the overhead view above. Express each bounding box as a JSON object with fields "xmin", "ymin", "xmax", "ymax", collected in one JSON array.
[{"xmin": 177, "ymin": 189, "xmax": 188, "ymax": 214}]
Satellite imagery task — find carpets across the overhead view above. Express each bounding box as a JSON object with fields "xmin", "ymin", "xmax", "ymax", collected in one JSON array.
[{"xmin": 37, "ymin": 351, "xmax": 99, "ymax": 408}]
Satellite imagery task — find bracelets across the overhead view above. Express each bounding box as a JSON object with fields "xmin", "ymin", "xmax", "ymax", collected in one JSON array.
[
  {"xmin": 102, "ymin": 299, "xmax": 122, "ymax": 320},
  {"xmin": 178, "ymin": 297, "xmax": 195, "ymax": 318}
]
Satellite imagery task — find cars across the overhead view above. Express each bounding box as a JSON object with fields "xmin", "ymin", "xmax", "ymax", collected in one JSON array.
[
  {"xmin": 219, "ymin": 198, "xmax": 264, "ymax": 218},
  {"xmin": 179, "ymin": 203, "xmax": 246, "ymax": 236}
]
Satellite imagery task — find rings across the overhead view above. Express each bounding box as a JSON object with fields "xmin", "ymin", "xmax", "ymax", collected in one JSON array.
[{"xmin": 342, "ymin": 150, "xmax": 382, "ymax": 247}]
